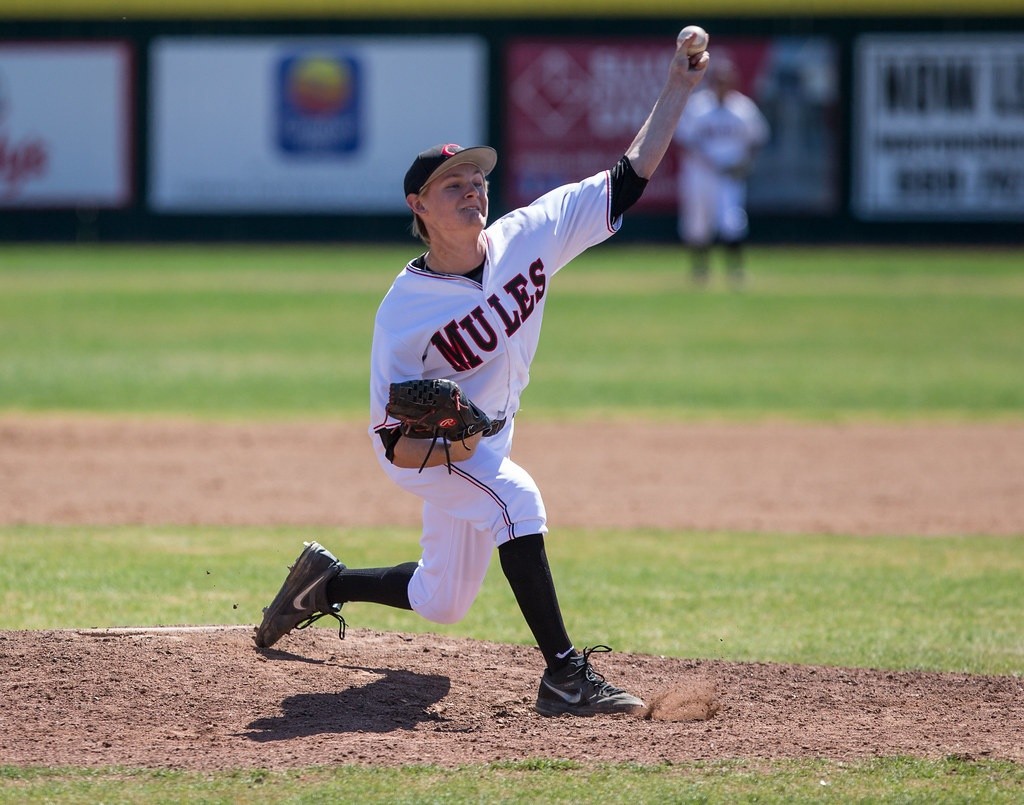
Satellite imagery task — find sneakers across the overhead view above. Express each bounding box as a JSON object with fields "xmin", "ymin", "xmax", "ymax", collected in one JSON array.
[
  {"xmin": 535, "ymin": 645, "xmax": 647, "ymax": 717},
  {"xmin": 251, "ymin": 540, "xmax": 349, "ymax": 650}
]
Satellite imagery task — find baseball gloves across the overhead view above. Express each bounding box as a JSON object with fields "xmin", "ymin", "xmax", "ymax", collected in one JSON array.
[{"xmin": 385, "ymin": 378, "xmax": 492, "ymax": 475}]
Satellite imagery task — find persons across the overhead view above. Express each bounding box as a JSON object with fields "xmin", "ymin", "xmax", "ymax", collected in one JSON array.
[
  {"xmin": 673, "ymin": 57, "xmax": 769, "ymax": 291},
  {"xmin": 253, "ymin": 34, "xmax": 712, "ymax": 714}
]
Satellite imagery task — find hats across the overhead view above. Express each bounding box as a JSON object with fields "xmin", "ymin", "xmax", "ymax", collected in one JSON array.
[{"xmin": 404, "ymin": 144, "xmax": 498, "ymax": 197}]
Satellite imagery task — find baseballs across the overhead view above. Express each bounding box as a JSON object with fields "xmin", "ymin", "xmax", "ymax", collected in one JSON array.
[{"xmin": 675, "ymin": 25, "xmax": 710, "ymax": 55}]
